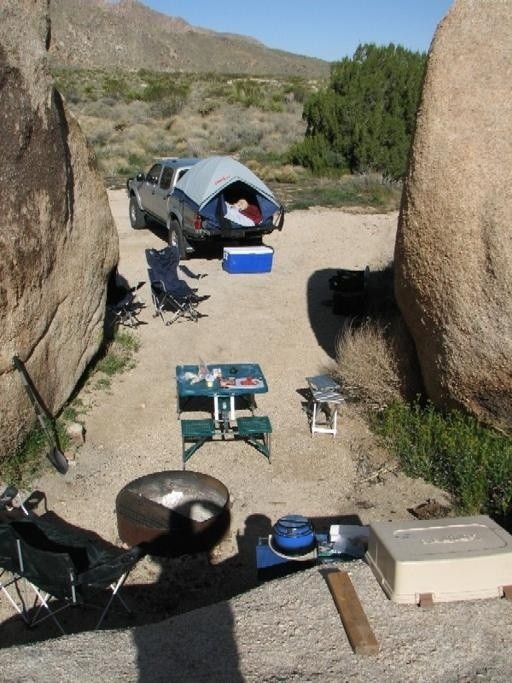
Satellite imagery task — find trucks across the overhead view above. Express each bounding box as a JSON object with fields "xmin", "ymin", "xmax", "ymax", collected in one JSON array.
[{"xmin": 128, "ymin": 159, "xmax": 284, "ymax": 260}]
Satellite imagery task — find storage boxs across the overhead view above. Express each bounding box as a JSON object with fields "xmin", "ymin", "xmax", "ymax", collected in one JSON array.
[
  {"xmin": 365, "ymin": 516, "xmax": 512, "ymax": 608},
  {"xmin": 222, "ymin": 246, "xmax": 274, "ymax": 274}
]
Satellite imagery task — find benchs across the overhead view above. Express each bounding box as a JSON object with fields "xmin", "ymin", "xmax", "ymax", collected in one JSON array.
[{"xmin": 181, "ymin": 416, "xmax": 272, "ymax": 470}]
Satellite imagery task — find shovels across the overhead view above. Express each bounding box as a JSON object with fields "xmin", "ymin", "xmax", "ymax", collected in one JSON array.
[{"xmin": 13, "ymin": 355, "xmax": 69, "ymax": 475}]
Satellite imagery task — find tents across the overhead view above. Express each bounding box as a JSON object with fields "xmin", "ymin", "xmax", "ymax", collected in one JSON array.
[{"xmin": 168, "ymin": 156, "xmax": 284, "ymax": 239}]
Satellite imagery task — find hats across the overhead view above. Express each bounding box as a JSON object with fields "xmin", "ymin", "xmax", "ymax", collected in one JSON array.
[{"xmin": 240, "ymin": 200, "xmax": 248, "ymax": 212}]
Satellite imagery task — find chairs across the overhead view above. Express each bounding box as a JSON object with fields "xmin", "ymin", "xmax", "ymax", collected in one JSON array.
[
  {"xmin": 145, "ymin": 246, "xmax": 204, "ymax": 326},
  {"xmin": 0, "ymin": 485, "xmax": 150, "ymax": 638},
  {"xmin": 107, "ymin": 273, "xmax": 147, "ymax": 329}
]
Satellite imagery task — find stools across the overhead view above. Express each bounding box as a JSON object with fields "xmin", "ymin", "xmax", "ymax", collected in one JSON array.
[
  {"xmin": 308, "ymin": 390, "xmax": 344, "ymax": 438},
  {"xmin": 301, "ymin": 374, "xmax": 340, "ymax": 417}
]
